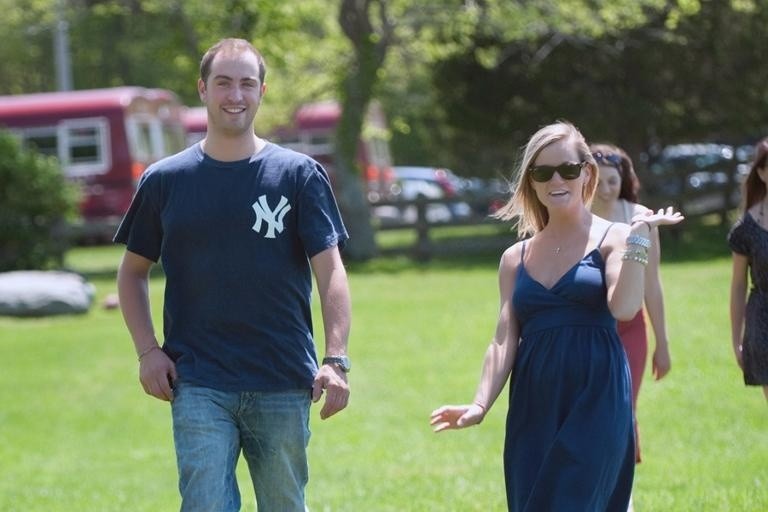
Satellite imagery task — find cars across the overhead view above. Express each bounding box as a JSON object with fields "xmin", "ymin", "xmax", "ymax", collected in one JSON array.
[
  {"xmin": 640, "ymin": 143, "xmax": 754, "ymax": 216},
  {"xmin": 375, "ymin": 166, "xmax": 509, "ymax": 226}
]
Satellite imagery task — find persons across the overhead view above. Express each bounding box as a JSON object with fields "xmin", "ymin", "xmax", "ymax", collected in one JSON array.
[
  {"xmin": 113, "ymin": 38, "xmax": 354, "ymax": 512},
  {"xmin": 427, "ymin": 119, "xmax": 686, "ymax": 512},
  {"xmin": 726, "ymin": 138, "xmax": 768, "ymax": 404},
  {"xmin": 584, "ymin": 143, "xmax": 672, "ymax": 512}
]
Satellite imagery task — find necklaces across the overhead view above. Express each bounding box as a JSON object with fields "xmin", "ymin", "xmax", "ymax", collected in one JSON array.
[{"xmin": 551, "ymin": 247, "xmax": 560, "ymax": 257}]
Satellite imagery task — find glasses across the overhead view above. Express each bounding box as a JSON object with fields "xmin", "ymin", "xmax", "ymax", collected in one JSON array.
[
  {"xmin": 592, "ymin": 151, "xmax": 621, "ymax": 162},
  {"xmin": 530, "ymin": 160, "xmax": 587, "ymax": 182}
]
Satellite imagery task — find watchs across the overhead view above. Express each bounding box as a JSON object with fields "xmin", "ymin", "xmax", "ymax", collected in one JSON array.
[{"xmin": 322, "ymin": 355, "xmax": 351, "ymax": 373}]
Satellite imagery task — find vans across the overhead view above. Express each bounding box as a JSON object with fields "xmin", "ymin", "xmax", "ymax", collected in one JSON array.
[
  {"xmin": 0, "ymin": 86, "xmax": 186, "ymax": 245},
  {"xmin": 180, "ymin": 97, "xmax": 391, "ymax": 202}
]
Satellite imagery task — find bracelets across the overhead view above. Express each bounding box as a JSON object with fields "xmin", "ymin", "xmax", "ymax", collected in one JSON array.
[
  {"xmin": 474, "ymin": 401, "xmax": 486, "ymax": 425},
  {"xmin": 620, "ymin": 248, "xmax": 648, "ymax": 260},
  {"xmin": 626, "ymin": 235, "xmax": 649, "ymax": 254},
  {"xmin": 137, "ymin": 345, "xmax": 161, "ymax": 362},
  {"xmin": 621, "ymin": 255, "xmax": 648, "ymax": 267},
  {"xmin": 631, "ymin": 219, "xmax": 651, "ymax": 232}
]
